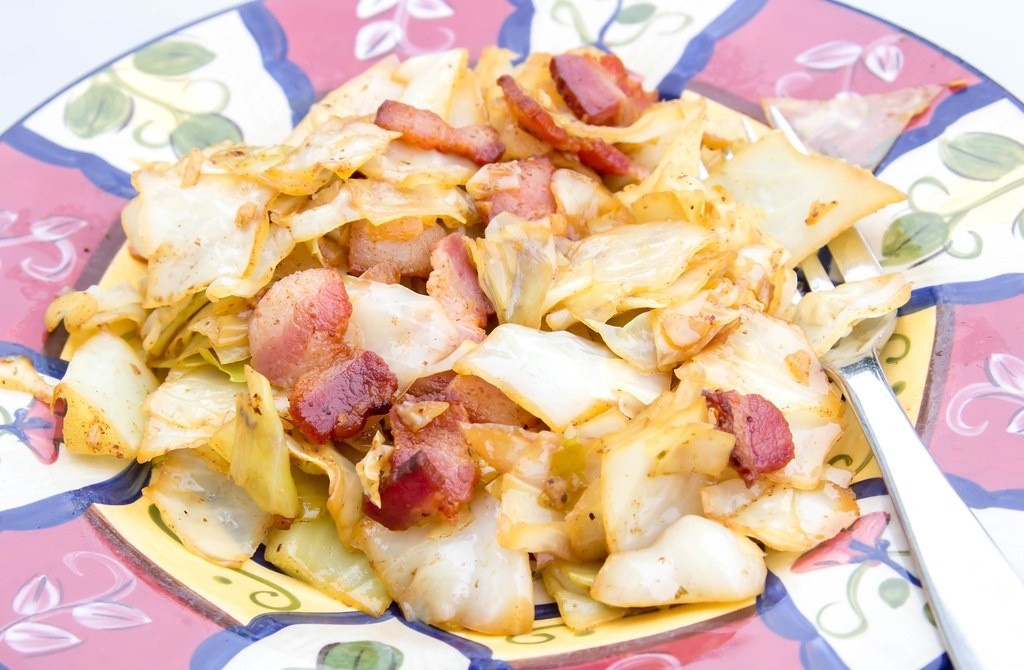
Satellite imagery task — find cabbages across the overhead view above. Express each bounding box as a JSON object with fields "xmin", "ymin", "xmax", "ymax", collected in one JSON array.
[{"xmin": 0, "ymin": 48, "xmax": 917, "ymax": 636}]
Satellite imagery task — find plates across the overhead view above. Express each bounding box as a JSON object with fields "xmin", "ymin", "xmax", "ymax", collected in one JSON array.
[{"xmin": 1, "ymin": 0, "xmax": 1024, "ymax": 669}]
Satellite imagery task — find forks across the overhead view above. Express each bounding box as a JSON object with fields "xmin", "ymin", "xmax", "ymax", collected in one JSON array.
[{"xmin": 691, "ymin": 105, "xmax": 1023, "ymax": 669}]
